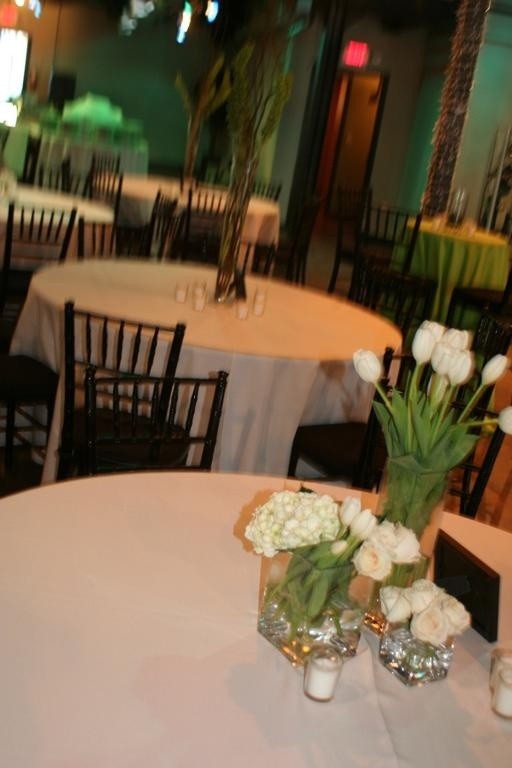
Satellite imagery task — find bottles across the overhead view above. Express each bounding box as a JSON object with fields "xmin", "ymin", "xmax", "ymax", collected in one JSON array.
[
  {"xmin": 175, "ymin": 280, "xmax": 268, "ymax": 319},
  {"xmin": 488, "ymin": 645, "xmax": 512, "ymax": 719},
  {"xmin": 304, "ymin": 642, "xmax": 342, "ymax": 703}
]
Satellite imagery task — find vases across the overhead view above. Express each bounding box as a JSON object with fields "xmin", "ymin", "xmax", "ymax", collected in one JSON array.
[
  {"xmin": 256, "ymin": 548, "xmax": 373, "ymax": 665},
  {"xmin": 360, "ymin": 457, "xmax": 451, "ymax": 633},
  {"xmin": 378, "ymin": 618, "xmax": 456, "ymax": 686}
]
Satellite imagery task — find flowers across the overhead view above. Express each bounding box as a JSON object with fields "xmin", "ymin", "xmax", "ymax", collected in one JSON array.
[
  {"xmin": 244, "ymin": 484, "xmax": 424, "ymax": 645},
  {"xmin": 377, "ymin": 577, "xmax": 471, "ymax": 647}
]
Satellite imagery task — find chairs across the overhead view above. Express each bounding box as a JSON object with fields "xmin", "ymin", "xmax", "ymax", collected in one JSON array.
[{"xmin": 0, "ymin": 126, "xmax": 512, "ymax": 521}]
